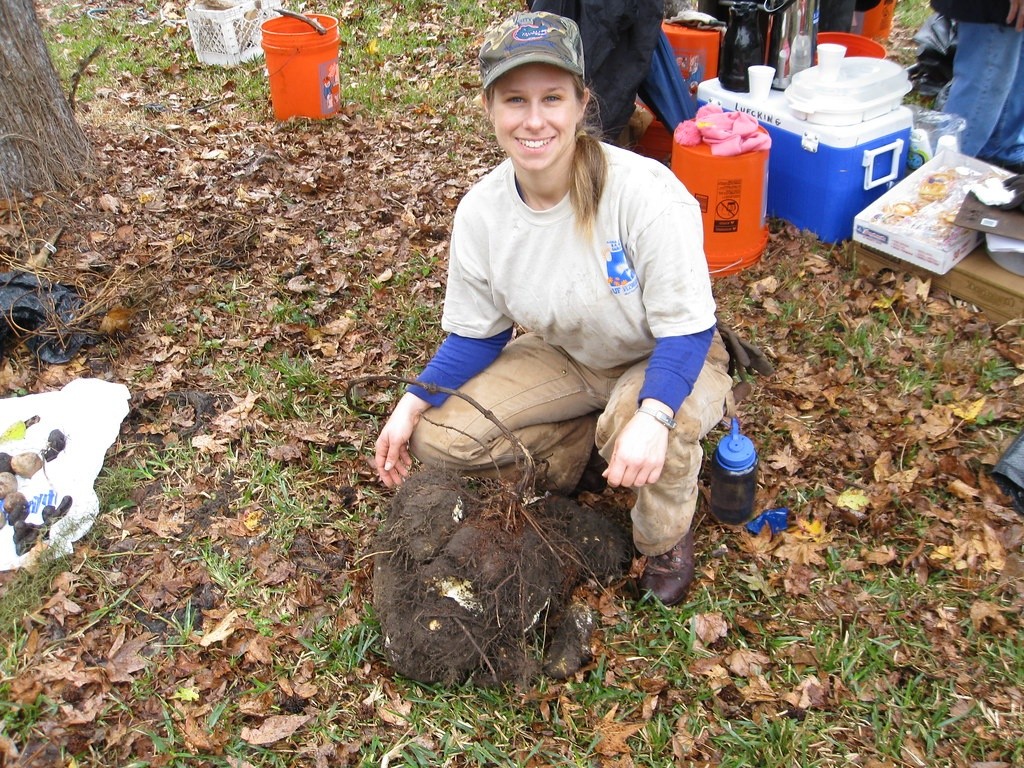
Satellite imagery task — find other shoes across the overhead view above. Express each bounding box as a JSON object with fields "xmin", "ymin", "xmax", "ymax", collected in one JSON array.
[
  {"xmin": 638, "ymin": 529, "xmax": 694, "ymax": 609},
  {"xmin": 567, "ymin": 460, "xmax": 608, "ymax": 498}
]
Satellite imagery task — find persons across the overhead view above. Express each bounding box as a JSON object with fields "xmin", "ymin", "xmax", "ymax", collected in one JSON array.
[
  {"xmin": 373, "ymin": 12, "xmax": 731, "ymax": 606},
  {"xmin": 929, "ymin": 1, "xmax": 1023, "ymax": 172}
]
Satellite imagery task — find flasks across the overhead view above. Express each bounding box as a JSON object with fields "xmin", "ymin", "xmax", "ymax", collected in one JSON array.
[{"xmin": 764, "ymin": 0, "xmax": 820, "ymax": 92}]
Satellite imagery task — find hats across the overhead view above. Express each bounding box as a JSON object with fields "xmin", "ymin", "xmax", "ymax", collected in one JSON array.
[{"xmin": 478, "ymin": 11, "xmax": 585, "ymax": 89}]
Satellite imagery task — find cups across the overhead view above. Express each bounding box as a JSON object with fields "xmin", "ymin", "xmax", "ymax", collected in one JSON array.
[
  {"xmin": 908, "ymin": 128, "xmax": 934, "ymax": 159},
  {"xmin": 816, "ymin": 42, "xmax": 847, "ymax": 79},
  {"xmin": 935, "ymin": 135, "xmax": 958, "ymax": 158},
  {"xmin": 747, "ymin": 65, "xmax": 776, "ymax": 100}
]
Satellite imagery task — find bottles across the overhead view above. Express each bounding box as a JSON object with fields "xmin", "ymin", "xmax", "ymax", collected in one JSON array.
[
  {"xmin": 709, "ymin": 416, "xmax": 758, "ymax": 524},
  {"xmin": 719, "ymin": 1, "xmax": 760, "ymax": 93}
]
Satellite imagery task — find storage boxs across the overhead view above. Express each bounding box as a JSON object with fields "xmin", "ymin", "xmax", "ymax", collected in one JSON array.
[
  {"xmin": 185, "ymin": 0, "xmax": 282, "ymax": 67},
  {"xmin": 697, "ymin": 77, "xmax": 914, "ymax": 244},
  {"xmin": 853, "ymin": 148, "xmax": 1021, "ymax": 275},
  {"xmin": 854, "ymin": 240, "xmax": 1024, "ymax": 337}
]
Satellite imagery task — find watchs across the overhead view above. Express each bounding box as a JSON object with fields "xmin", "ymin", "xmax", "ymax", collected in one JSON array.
[{"xmin": 637, "ymin": 406, "xmax": 678, "ymax": 430}]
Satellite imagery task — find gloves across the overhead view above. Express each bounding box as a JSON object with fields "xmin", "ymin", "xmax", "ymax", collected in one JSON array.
[{"xmin": 717, "ymin": 320, "xmax": 774, "ymax": 383}]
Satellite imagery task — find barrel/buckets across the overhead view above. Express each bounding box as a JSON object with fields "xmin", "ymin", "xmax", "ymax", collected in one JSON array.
[
  {"xmin": 862, "ymin": 0, "xmax": 897, "ymax": 41},
  {"xmin": 632, "ymin": 20, "xmax": 720, "ymax": 161},
  {"xmin": 259, "ymin": 13, "xmax": 341, "ymax": 122},
  {"xmin": 671, "ymin": 117, "xmax": 772, "ymax": 278},
  {"xmin": 814, "ymin": 32, "xmax": 888, "ymax": 66}
]
